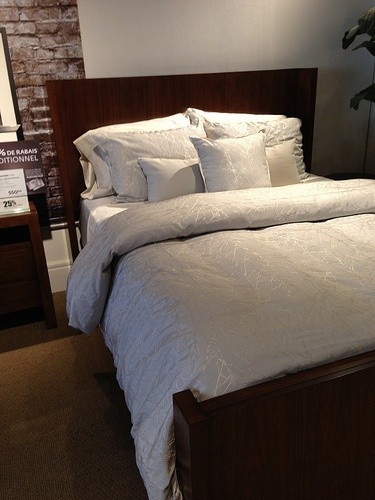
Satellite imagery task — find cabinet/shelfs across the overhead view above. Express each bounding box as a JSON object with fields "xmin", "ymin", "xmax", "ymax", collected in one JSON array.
[{"xmin": 2, "ymin": 206, "xmax": 60, "ymax": 329}]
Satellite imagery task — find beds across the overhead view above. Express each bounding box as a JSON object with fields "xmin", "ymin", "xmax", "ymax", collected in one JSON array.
[{"xmin": 43, "ymin": 67, "xmax": 375, "ymax": 499}]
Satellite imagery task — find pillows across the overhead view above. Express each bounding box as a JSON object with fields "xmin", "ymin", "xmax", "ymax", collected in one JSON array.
[
  {"xmin": 190, "ymin": 128, "xmax": 274, "ymax": 196},
  {"xmin": 264, "ymin": 137, "xmax": 305, "ymax": 188},
  {"xmin": 74, "ymin": 110, "xmax": 194, "ymax": 197},
  {"xmin": 201, "ymin": 117, "xmax": 311, "ymax": 185},
  {"xmin": 88, "ymin": 124, "xmax": 207, "ymax": 204},
  {"xmin": 136, "ymin": 155, "xmax": 206, "ymax": 202},
  {"xmin": 189, "ymin": 102, "xmax": 289, "ymax": 127}
]
